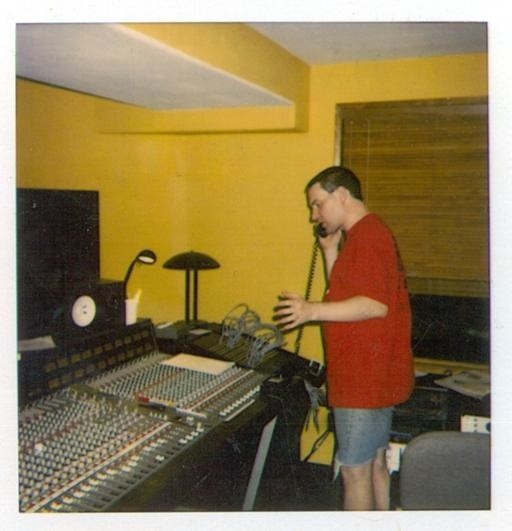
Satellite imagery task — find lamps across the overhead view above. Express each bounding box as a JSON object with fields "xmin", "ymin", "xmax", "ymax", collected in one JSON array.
[{"xmin": 123, "ymin": 249, "xmax": 157, "ymax": 296}]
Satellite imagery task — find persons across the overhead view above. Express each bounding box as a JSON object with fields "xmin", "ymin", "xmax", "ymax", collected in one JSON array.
[{"xmin": 271, "ymin": 164, "xmax": 417, "ymax": 510}]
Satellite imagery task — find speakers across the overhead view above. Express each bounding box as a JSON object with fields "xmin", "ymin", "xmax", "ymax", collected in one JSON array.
[{"xmin": 65, "ymin": 279, "xmax": 125, "ymax": 337}]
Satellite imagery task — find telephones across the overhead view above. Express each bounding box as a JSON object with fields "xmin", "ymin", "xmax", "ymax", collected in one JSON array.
[{"xmin": 316, "ymin": 222, "xmax": 327, "ymax": 238}]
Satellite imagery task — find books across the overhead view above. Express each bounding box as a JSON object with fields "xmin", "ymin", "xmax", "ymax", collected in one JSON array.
[{"xmin": 434, "ymin": 370, "xmax": 490, "ymax": 403}]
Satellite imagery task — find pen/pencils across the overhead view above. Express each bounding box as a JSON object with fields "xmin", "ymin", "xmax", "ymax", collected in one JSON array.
[{"xmin": 137, "ymin": 395, "xmax": 208, "ymax": 419}]
